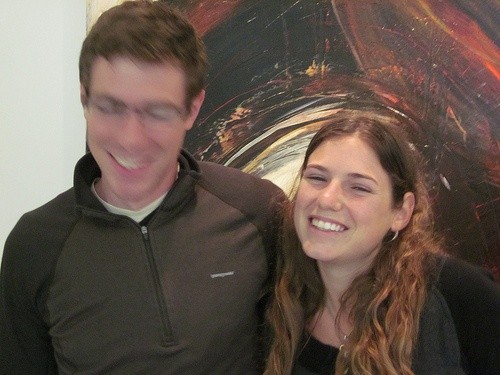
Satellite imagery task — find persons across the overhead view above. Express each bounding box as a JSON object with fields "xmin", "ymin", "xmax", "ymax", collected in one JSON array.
[
  {"xmin": 259, "ymin": 113, "xmax": 500, "ymax": 375},
  {"xmin": 0, "ymin": 1, "xmax": 291, "ymax": 375}
]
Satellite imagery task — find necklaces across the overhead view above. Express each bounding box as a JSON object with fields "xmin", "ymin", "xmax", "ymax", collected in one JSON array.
[{"xmin": 322, "ymin": 305, "xmax": 360, "ymax": 354}]
